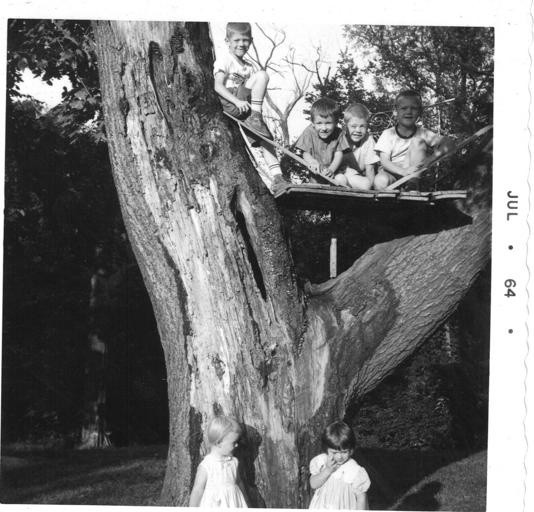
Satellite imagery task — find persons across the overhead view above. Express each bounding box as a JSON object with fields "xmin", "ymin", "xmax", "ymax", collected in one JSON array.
[
  {"xmin": 188, "ymin": 415, "xmax": 248, "ymax": 507},
  {"xmin": 293, "ymin": 98, "xmax": 371, "ymax": 192},
  {"xmin": 343, "ymin": 103, "xmax": 380, "ymax": 187},
  {"xmin": 307, "ymin": 420, "xmax": 372, "ymax": 509},
  {"xmin": 372, "ymin": 90, "xmax": 454, "ymax": 191},
  {"xmin": 212, "ymin": 22, "xmax": 294, "ymax": 193}
]
